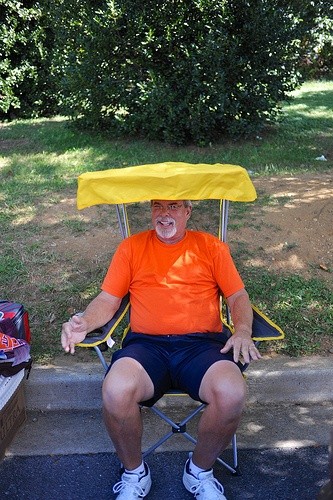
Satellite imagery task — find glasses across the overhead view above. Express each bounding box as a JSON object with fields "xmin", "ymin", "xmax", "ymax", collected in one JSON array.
[{"xmin": 150, "ymin": 204, "xmax": 182, "ymax": 211}]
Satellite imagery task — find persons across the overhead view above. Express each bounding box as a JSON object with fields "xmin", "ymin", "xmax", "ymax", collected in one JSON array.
[{"xmin": 60, "ymin": 200, "xmax": 262, "ymax": 500}]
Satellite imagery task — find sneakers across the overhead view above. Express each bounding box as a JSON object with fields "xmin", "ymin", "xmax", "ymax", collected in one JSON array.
[
  {"xmin": 112, "ymin": 461, "xmax": 151, "ymax": 500},
  {"xmin": 182, "ymin": 452, "xmax": 227, "ymax": 500}
]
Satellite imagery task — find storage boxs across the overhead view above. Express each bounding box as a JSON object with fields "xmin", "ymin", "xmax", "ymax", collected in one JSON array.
[{"xmin": 0, "ymin": 368, "xmax": 27, "ymax": 464}]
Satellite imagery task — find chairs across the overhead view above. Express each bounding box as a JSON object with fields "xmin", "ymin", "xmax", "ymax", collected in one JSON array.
[{"xmin": 73, "ymin": 162, "xmax": 284, "ymax": 476}]
